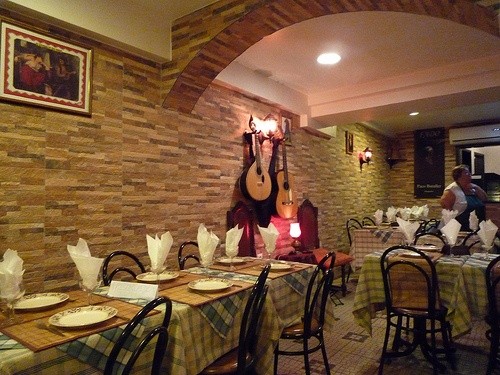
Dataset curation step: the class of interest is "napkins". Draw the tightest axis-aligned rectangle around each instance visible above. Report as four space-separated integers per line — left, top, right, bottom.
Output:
371 203 499 249
225 223 244 256
257 222 279 252
145 231 173 271
66 239 105 290
197 223 220 266
0 248 25 299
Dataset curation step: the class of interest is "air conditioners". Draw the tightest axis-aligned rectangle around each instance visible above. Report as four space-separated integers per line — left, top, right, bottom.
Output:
448 124 500 146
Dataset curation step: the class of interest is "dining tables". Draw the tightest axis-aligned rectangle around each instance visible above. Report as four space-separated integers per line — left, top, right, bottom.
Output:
0 222 500 375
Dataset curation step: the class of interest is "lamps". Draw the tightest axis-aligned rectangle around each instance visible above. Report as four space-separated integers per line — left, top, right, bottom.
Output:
289 223 305 254
359 147 373 170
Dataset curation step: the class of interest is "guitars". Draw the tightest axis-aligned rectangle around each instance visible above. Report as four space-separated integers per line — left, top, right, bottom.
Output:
238 122 273 205
271 124 299 221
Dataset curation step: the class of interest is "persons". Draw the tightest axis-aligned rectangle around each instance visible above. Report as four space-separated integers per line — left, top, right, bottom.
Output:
439 165 487 232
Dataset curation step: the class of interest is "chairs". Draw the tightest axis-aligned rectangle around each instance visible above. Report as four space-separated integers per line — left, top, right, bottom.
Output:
101 214 500 375
296 200 353 297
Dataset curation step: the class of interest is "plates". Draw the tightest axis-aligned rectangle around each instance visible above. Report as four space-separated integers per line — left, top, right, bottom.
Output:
7 292 70 309
261 263 291 270
216 256 244 263
187 277 234 292
48 305 119 327
400 244 444 258
136 271 179 282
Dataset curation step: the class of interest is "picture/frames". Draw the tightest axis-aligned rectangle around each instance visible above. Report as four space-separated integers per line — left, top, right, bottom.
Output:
346 130 354 155
279 113 293 146
0 17 94 119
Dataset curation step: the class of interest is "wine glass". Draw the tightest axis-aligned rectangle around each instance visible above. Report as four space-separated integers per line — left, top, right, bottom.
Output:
445 239 458 259
480 240 494 261
403 234 415 250
